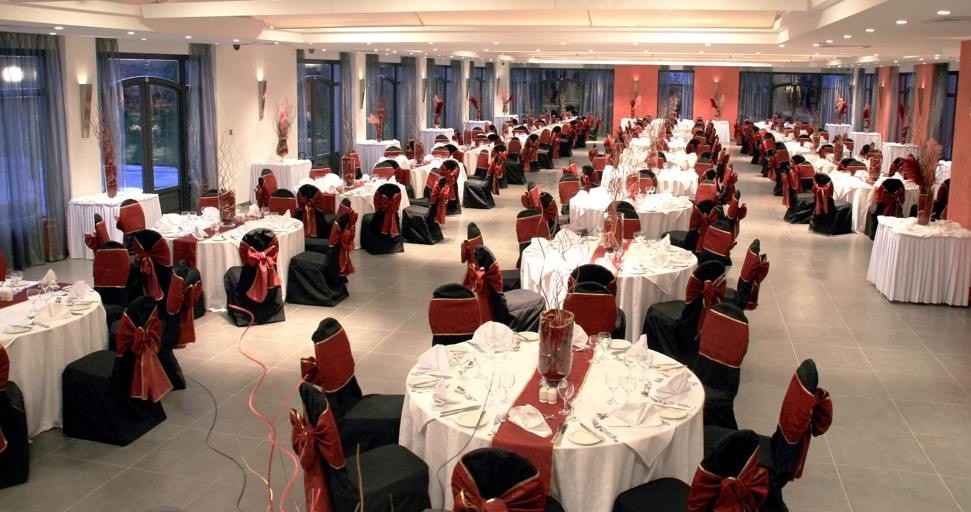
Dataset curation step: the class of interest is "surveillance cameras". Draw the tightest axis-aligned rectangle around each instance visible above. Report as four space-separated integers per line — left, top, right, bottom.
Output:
233 45 240 50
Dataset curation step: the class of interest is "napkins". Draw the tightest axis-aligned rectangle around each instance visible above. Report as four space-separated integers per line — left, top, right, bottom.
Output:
623 334 651 361
655 370 692 394
66 280 90 300
415 344 451 376
472 321 515 350
572 321 588 346
34 302 70 324
607 399 664 428
432 377 463 406
39 268 57 287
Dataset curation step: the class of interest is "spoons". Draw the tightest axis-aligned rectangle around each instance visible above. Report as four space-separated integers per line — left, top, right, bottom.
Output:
457 360 474 376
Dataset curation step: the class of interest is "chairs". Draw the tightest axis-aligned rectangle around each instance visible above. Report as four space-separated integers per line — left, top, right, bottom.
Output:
724 237 769 312
683 303 750 429
704 358 833 511
463 245 547 332
428 282 483 348
285 212 356 307
83 213 110 248
641 261 728 367
105 228 187 391
562 281 618 339
300 316 406 459
611 429 768 512
92 240 131 313
226 109 749 280
0 346 31 490
289 381 433 512
565 263 627 340
59 295 174 448
733 113 952 243
114 199 146 235
450 446 567 512
223 228 287 328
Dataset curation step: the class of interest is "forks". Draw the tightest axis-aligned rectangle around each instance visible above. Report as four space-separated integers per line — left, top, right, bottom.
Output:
592 418 618 438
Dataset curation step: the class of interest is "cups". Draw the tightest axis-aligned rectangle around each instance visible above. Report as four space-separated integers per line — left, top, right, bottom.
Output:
539 387 549 403
549 389 558 404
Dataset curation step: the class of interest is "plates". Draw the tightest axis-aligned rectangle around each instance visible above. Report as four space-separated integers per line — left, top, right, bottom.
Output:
409 376 439 388
445 343 468 357
69 305 91 311
609 338 632 349
516 331 540 341
455 411 489 428
6 327 31 333
568 428 603 445
660 407 688 418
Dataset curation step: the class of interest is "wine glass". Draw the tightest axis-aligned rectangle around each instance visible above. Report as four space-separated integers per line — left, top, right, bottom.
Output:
589 334 601 364
623 375 636 400
484 354 518 404
646 186 656 198
5 271 23 295
558 380 575 414
604 374 623 405
597 332 612 352
182 210 197 226
26 289 40 312
614 247 624 260
568 395 584 423
254 207 278 226
634 231 646 245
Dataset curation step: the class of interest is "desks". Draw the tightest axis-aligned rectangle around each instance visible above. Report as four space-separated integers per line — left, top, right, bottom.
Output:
520 235 698 342
1 280 109 440
398 333 706 512
151 211 305 312
865 215 971 306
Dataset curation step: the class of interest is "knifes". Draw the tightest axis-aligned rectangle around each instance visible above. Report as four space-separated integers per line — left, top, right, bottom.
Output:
581 423 605 440
